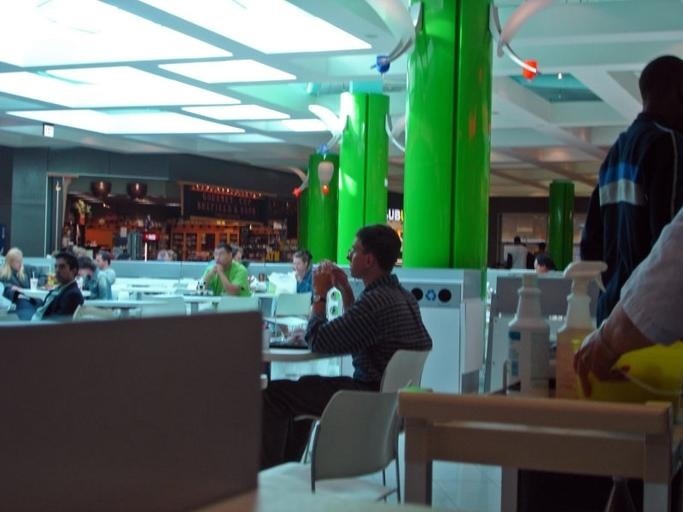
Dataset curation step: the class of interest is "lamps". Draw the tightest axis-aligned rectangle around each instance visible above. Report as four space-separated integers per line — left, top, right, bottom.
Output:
385 112 405 153
308 103 347 160
489 0 552 80
370 1 423 75
290 167 308 197
317 162 334 195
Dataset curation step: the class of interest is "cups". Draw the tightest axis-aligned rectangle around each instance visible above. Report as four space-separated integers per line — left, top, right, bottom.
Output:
30 278 38 291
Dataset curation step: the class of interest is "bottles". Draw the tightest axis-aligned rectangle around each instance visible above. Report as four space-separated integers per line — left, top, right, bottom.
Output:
556 278 597 400
258 297 272 316
47 276 54 291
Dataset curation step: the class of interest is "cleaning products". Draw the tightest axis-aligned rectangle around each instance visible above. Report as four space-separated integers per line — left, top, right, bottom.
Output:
551 253 606 398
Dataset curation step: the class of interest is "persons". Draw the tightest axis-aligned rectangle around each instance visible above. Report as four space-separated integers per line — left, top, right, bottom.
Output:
573 206 683 397
532 253 558 275
505 235 546 270
0 223 117 322
579 54 683 331
259 223 433 472
230 244 244 263
201 242 252 298
291 248 315 295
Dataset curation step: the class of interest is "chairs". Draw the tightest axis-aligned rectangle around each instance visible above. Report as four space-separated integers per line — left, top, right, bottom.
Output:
398 390 672 512
